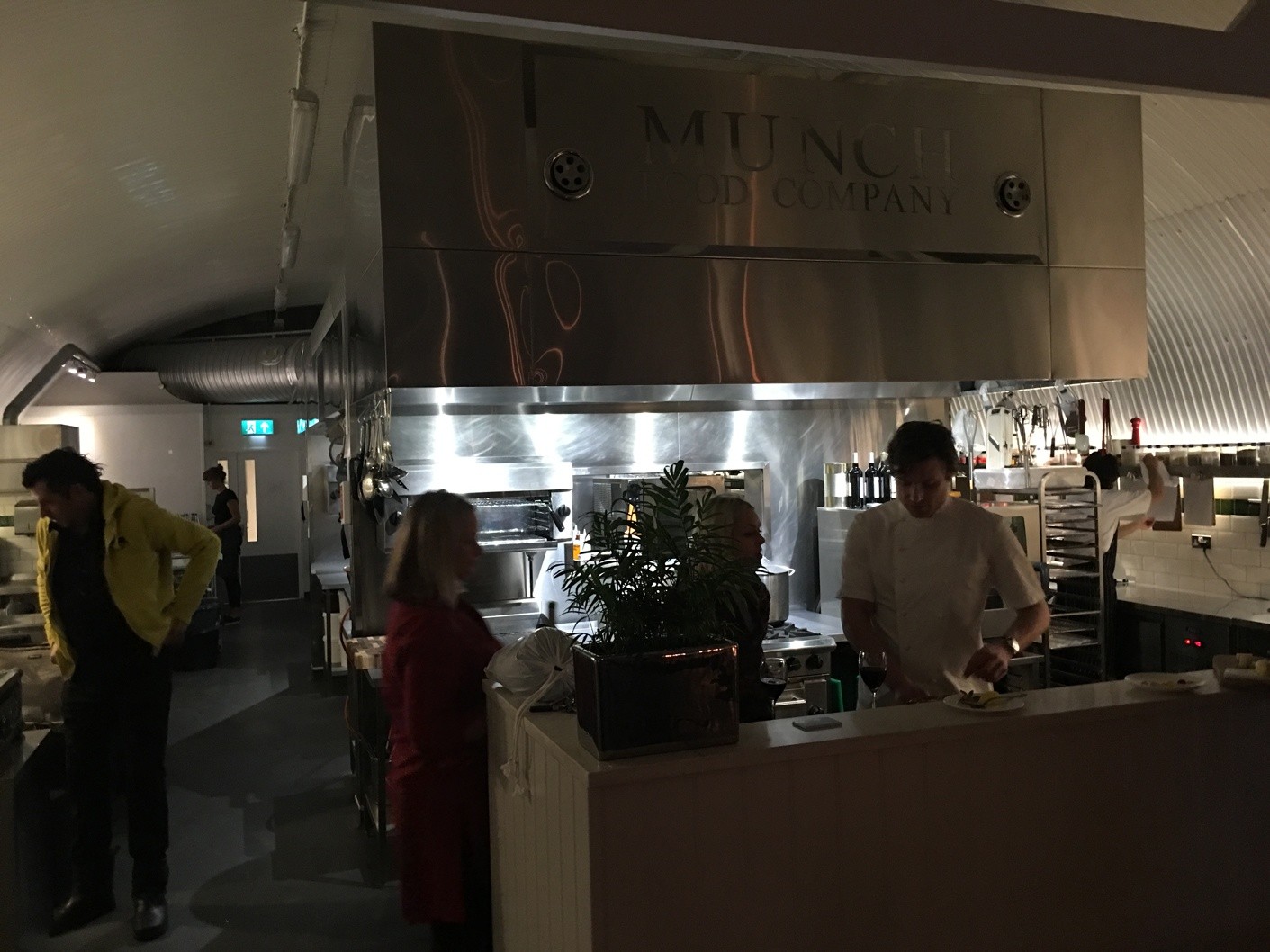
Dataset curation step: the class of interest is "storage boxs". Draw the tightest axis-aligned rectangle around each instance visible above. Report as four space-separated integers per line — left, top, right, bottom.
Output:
1028 464 1087 488
973 468 1026 490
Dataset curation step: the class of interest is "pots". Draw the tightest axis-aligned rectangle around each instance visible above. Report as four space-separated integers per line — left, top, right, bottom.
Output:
756 565 795 622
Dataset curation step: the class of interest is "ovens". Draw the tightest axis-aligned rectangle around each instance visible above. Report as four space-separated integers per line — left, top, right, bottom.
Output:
771 680 828 719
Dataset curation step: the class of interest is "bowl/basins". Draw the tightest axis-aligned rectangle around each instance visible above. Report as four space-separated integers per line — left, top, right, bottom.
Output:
343 565 351 584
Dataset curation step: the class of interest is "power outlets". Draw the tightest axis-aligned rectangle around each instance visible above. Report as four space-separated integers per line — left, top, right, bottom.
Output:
1191 535 1212 549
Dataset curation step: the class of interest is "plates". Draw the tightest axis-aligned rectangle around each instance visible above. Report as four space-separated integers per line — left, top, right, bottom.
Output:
943 693 1024 712
1124 672 1211 692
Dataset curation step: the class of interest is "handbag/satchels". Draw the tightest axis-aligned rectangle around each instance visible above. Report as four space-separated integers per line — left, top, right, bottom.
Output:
484 626 583 796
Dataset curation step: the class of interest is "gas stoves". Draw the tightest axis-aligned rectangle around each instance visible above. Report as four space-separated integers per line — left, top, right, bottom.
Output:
761 622 837 678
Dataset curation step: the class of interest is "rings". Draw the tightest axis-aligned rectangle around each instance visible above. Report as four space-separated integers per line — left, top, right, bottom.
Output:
909 699 912 702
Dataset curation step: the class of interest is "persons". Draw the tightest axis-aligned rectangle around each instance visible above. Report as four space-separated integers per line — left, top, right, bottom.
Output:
1010 449 1034 466
202 464 243 626
22 447 222 942
380 491 503 952
1052 449 1165 686
835 421 1050 711
668 495 776 723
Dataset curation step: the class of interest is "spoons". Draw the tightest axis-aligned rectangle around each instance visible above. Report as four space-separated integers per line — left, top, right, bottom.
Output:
967 693 1028 709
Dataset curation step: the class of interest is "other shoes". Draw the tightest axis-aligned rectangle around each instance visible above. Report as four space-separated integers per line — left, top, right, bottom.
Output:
133 892 168 940
220 615 240 627
52 887 115 929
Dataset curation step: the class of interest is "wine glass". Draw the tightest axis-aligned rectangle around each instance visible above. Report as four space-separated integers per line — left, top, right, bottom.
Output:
858 649 888 710
759 657 787 720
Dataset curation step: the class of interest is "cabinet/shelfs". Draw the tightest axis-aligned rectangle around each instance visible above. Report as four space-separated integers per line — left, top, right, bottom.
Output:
970 471 1107 690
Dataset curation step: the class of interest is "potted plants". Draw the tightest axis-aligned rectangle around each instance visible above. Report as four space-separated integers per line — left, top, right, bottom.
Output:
546 459 776 760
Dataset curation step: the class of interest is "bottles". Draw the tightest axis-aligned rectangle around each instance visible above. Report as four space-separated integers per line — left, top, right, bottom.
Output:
848 452 896 510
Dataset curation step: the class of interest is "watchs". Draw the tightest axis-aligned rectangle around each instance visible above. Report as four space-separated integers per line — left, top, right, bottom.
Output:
1003 635 1022 657
